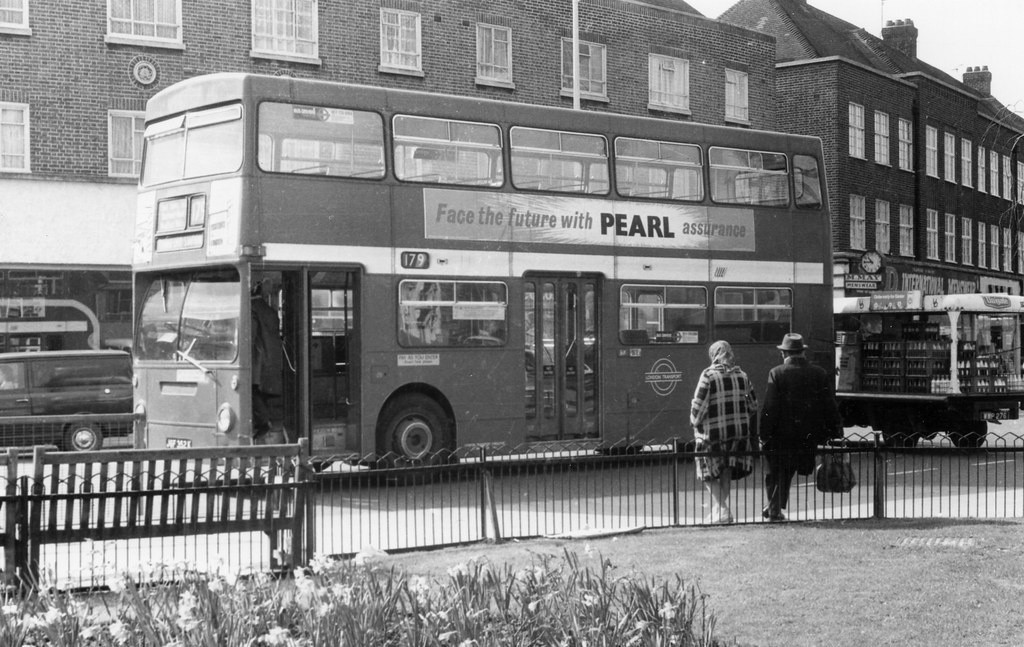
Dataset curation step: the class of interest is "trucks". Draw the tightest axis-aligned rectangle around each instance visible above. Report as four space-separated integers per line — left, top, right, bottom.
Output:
829 294 1024 451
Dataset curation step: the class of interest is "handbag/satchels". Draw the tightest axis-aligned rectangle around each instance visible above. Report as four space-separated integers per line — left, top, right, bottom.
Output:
815 451 857 493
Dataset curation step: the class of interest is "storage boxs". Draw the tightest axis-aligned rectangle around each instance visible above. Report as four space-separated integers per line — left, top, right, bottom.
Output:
862 341 1024 393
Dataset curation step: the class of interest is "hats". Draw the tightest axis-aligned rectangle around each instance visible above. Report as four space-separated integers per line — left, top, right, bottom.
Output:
776 333 809 351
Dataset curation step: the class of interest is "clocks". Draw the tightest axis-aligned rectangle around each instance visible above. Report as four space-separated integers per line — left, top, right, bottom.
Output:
860 251 886 274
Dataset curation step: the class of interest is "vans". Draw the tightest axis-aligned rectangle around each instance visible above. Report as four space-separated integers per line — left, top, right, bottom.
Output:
0 349 132 451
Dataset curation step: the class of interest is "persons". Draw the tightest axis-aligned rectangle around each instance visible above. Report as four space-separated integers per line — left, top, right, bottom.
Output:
759 333 828 521
689 339 757 524
248 278 285 445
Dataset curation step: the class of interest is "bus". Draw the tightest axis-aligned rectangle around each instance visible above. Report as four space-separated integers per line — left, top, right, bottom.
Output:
132 71 835 483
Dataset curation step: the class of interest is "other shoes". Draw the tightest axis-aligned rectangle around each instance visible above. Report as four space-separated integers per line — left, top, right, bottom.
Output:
253 422 272 440
762 503 784 520
709 506 733 524
